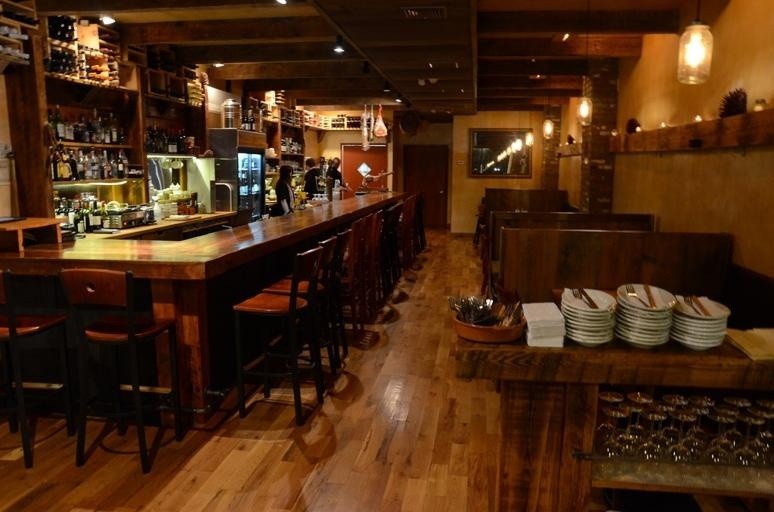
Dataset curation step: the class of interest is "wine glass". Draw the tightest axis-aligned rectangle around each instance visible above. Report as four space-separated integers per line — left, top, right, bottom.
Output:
312 193 328 202
595 391 774 492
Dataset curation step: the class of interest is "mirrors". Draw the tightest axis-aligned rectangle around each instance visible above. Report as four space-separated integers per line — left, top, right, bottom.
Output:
469 127 534 179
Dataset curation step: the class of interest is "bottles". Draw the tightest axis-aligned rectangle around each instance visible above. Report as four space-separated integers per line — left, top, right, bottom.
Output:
243 105 256 131
47 103 119 145
331 116 362 128
53 192 103 234
145 121 187 152
260 103 303 127
48 145 129 182
44 15 120 82
186 82 206 103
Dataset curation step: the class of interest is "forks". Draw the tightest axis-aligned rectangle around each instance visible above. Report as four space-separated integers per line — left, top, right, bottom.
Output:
445 290 496 323
684 294 702 316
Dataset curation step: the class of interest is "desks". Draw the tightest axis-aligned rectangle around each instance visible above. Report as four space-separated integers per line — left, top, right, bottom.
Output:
93 208 237 239
453 328 773 512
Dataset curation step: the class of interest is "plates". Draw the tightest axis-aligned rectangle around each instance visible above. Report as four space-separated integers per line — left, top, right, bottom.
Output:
615 284 677 350
559 288 617 347
670 299 732 350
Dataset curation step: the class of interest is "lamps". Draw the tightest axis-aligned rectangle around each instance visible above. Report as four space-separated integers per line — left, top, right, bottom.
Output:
526 131 534 147
575 94 594 126
543 118 555 139
676 9 714 88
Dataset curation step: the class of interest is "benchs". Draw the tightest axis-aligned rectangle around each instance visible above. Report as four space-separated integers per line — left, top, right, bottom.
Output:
500 229 735 329
474 186 654 290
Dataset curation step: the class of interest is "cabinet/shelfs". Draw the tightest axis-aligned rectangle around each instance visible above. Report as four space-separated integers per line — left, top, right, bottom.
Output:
275 103 305 170
0 17 149 251
141 51 207 156
304 109 383 132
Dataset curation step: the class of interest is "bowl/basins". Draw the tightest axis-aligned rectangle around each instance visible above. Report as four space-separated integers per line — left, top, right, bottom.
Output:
451 313 526 344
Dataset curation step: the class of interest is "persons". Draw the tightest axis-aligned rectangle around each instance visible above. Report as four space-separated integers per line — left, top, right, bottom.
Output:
326 158 352 200
303 158 323 200
275 165 296 216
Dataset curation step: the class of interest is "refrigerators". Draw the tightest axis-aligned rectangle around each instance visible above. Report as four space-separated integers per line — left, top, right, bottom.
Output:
206 127 266 227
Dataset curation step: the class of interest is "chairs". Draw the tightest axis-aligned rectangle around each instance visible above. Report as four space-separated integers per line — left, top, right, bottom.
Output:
57 267 187 473
0 268 79 469
